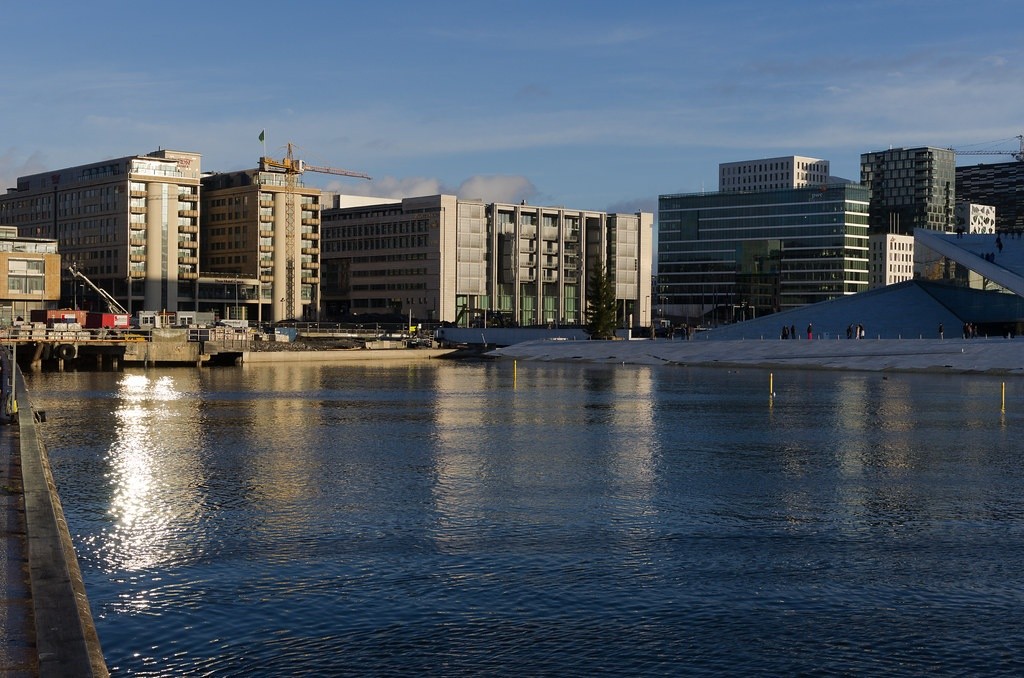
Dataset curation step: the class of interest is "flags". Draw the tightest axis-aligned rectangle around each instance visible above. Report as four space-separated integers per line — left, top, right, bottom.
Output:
259 130 264 142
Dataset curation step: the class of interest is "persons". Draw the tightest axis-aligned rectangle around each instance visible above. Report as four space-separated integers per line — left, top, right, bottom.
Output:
679 326 690 340
847 323 864 339
650 325 654 339
791 325 795 338
939 323 944 339
782 326 789 339
1002 324 1015 338
1011 229 1015 239
1018 229 1021 237
985 252 995 262
963 322 978 339
955 227 963 239
665 327 674 340
807 323 813 339
996 229 1003 253
1004 229 1008 238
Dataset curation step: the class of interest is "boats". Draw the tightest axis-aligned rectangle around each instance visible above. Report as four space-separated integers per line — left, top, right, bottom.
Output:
433 305 594 350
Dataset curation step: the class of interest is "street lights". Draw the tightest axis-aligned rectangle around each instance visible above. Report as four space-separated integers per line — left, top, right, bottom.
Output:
644 295 651 328
72 261 78 311
235 274 240 321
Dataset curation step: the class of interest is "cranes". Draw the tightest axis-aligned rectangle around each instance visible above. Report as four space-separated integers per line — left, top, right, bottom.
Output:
256 141 373 321
